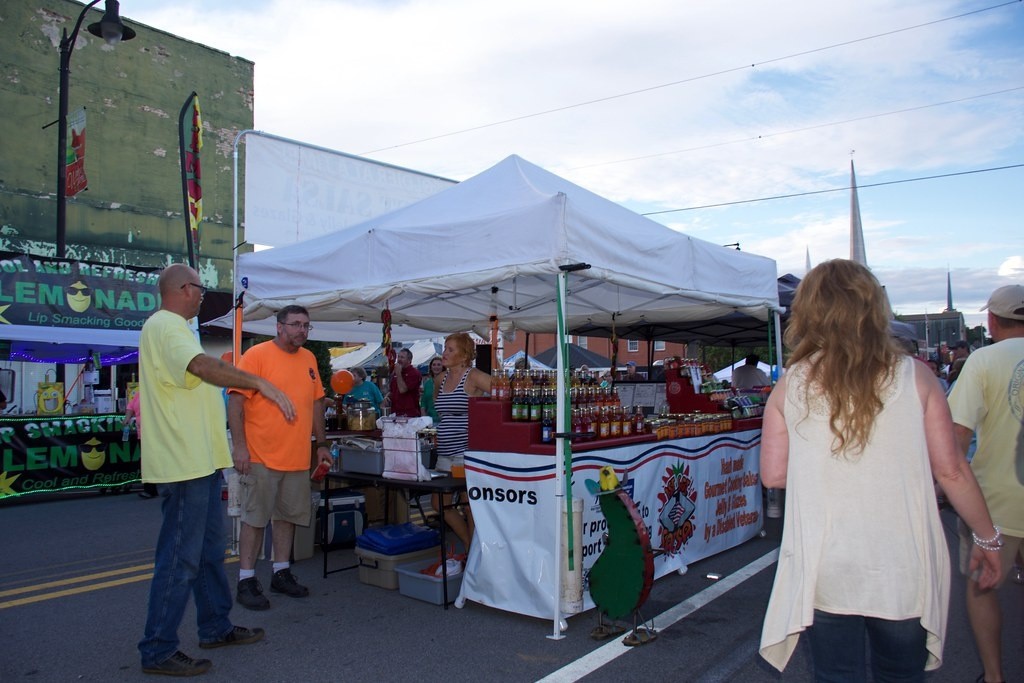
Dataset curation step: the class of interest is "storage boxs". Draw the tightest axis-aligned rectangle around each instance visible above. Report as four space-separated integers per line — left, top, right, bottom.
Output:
317 487 367 552
354 541 448 590
339 445 383 475
393 555 463 606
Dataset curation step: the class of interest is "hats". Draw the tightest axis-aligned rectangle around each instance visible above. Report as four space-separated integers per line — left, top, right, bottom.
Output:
979 284 1024 320
948 340 969 350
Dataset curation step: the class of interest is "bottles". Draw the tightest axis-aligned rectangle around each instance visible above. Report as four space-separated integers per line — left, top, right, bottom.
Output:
310 460 330 483
329 441 340 473
490 368 632 445
329 394 372 430
121 423 129 441
634 404 644 435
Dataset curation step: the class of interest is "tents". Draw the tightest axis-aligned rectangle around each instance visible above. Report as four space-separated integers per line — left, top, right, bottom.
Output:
570 274 917 380
228 154 785 639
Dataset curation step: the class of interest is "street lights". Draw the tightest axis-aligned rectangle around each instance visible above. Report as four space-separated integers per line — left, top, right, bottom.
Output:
937 326 956 373
54 0 136 417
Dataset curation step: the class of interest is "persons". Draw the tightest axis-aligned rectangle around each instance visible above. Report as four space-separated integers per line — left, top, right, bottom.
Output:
893 333 988 498
760 259 1002 683
122 263 529 674
732 354 771 400
947 285 1024 683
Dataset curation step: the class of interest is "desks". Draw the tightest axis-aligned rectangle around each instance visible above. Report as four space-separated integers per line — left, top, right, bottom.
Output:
322 472 469 611
455 396 769 630
312 429 382 469
0 414 144 507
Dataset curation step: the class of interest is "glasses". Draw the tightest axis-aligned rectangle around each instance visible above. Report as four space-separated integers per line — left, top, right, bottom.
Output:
180 283 207 295
278 321 313 330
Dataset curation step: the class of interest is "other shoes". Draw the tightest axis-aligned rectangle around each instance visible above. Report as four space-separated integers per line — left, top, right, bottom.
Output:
236 575 270 610
270 568 308 597
143 651 212 674
137 491 159 499
199 625 266 648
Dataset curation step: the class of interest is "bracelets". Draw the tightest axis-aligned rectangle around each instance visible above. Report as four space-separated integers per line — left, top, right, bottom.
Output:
971 524 1004 550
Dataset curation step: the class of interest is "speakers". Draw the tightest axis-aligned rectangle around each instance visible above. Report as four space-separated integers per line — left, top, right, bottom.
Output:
476 344 491 375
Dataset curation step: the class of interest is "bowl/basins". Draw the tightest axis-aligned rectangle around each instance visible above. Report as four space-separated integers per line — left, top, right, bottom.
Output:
345 407 377 430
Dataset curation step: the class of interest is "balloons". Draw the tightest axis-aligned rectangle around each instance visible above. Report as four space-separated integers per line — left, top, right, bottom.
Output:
331 370 355 394
